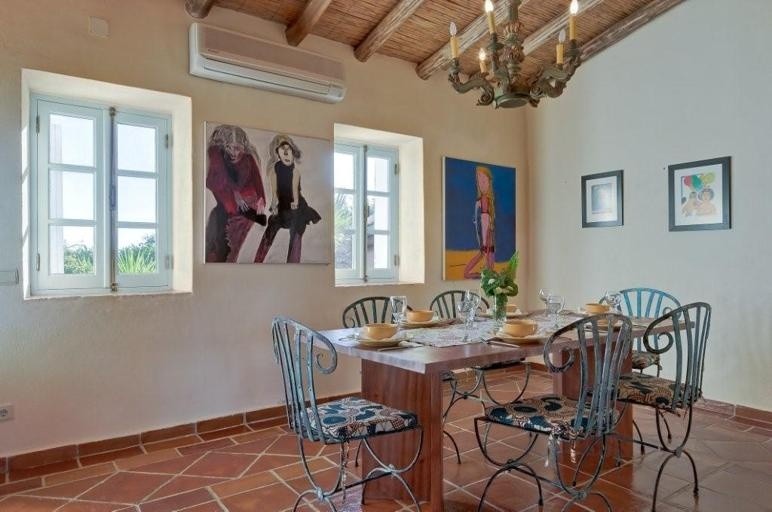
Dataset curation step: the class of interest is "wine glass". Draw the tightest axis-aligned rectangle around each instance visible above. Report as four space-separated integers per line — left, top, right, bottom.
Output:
538 289 565 331
603 289 624 319
456 286 481 342
390 296 407 339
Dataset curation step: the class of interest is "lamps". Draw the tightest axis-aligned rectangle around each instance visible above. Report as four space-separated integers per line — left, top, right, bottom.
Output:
447 0 581 109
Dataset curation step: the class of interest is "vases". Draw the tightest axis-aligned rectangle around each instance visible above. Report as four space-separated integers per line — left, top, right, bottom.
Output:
494 295 506 322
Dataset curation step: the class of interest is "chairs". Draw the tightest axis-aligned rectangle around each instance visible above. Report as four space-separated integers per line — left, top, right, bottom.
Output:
271 314 424 512
474 314 634 512
430 291 532 455
599 287 682 454
573 302 712 512
342 297 461 467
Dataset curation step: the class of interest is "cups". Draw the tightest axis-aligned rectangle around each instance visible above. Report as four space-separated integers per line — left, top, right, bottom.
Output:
406 310 435 321
363 324 397 339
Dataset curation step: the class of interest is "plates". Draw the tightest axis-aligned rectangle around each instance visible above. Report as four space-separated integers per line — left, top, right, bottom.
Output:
501 337 546 344
393 318 444 327
353 337 404 348
586 323 639 331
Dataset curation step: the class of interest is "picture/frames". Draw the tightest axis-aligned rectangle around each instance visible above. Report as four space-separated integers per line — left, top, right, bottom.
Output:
581 169 623 228
668 156 730 232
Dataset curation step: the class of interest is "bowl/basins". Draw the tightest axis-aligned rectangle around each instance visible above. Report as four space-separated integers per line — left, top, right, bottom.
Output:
504 305 517 312
504 320 538 337
585 303 604 314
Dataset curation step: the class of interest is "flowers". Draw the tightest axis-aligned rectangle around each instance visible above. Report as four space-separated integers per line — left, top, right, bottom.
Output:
480 252 520 319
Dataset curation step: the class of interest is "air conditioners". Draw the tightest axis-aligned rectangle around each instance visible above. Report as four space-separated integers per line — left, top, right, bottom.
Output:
188 22 347 104
